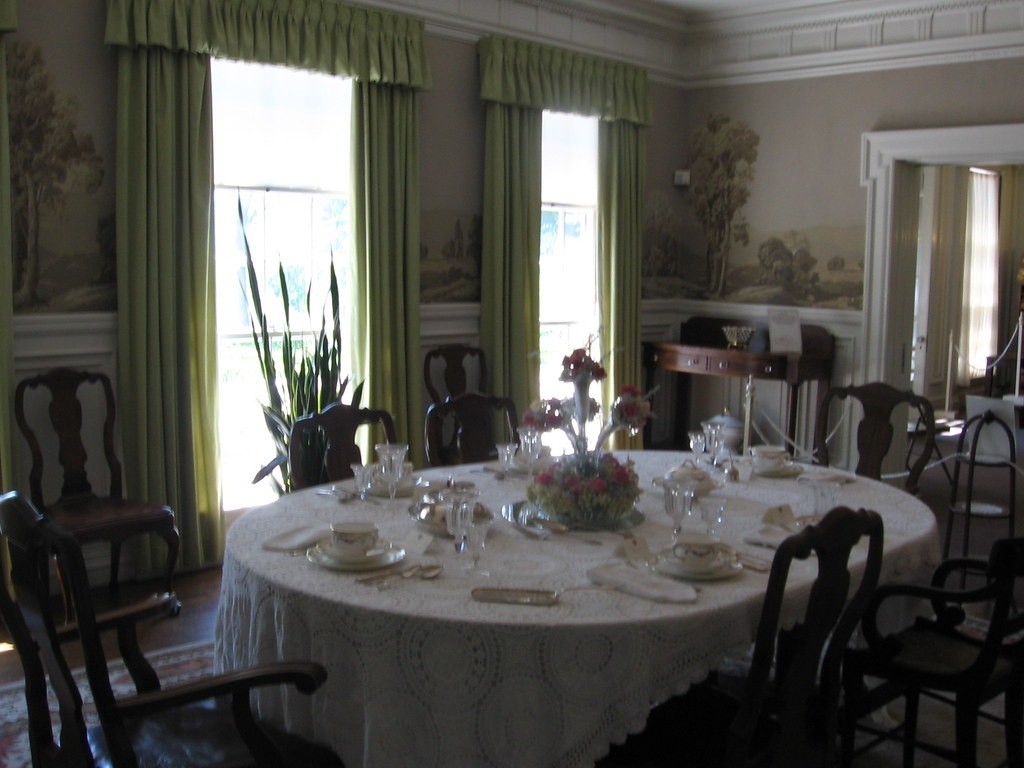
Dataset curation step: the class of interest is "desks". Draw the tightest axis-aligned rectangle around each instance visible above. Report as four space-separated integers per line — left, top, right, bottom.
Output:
641 318 836 453
220 450 943 767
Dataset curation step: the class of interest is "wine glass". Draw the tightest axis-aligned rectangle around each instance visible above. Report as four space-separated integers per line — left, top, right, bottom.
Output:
689 430 705 465
489 444 518 488
438 488 490 579
349 463 376 501
516 426 542 483
660 480 695 544
701 422 727 473
697 497 726 533
374 443 409 499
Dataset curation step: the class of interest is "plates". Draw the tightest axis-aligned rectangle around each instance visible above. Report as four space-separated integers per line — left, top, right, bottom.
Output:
315 538 392 564
307 544 405 570
753 465 804 477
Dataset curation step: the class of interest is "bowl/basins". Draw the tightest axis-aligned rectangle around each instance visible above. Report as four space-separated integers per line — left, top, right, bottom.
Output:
332 527 378 556
407 505 494 538
651 477 723 499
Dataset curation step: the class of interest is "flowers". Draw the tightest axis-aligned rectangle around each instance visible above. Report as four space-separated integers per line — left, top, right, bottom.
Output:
523 349 654 499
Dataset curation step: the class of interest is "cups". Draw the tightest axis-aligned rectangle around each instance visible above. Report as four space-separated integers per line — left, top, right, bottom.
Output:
751 446 790 471
722 326 754 351
647 547 742 580
795 480 840 529
708 406 744 448
671 534 720 565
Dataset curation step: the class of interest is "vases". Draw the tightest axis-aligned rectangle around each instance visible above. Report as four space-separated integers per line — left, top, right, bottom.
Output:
528 490 636 531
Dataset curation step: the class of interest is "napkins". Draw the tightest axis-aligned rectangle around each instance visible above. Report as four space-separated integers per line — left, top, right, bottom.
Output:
261 519 333 553
736 522 794 546
581 557 699 605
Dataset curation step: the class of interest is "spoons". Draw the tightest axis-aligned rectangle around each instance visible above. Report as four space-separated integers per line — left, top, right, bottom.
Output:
355 564 443 588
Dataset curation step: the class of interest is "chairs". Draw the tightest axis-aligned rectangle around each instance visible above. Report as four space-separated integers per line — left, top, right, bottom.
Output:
644 506 886 767
0 496 346 768
814 380 935 497
14 367 184 627
905 407 952 479
849 536 1024 768
423 392 523 469
290 402 396 492
423 341 487 407
